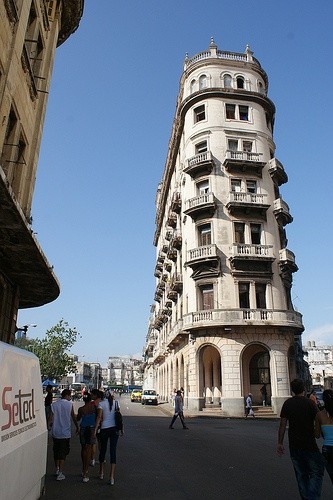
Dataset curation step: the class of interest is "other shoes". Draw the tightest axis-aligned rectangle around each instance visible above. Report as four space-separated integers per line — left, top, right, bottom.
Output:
169 426 175 429
56 474 66 480
108 479 114 485
83 477 90 483
56 469 60 475
183 426 190 429
99 473 104 479
90 459 96 467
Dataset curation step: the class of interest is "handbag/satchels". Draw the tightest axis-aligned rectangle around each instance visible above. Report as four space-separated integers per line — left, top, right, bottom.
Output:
250 409 255 416
115 401 123 430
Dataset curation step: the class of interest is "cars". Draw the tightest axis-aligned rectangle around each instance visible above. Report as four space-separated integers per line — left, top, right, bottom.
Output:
129 389 142 402
140 388 159 405
314 392 325 407
313 388 321 395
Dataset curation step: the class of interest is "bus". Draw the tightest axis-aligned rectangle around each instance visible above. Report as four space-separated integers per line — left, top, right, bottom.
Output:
70 382 87 397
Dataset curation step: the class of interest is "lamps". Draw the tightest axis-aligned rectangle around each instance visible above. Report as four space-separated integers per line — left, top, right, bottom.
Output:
15 324 28 335
225 328 232 330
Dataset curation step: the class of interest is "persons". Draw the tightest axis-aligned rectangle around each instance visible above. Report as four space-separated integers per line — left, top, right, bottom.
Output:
93 389 124 485
75 391 98 482
277 379 324 500
47 389 78 481
315 389 333 486
260 382 269 406
91 389 107 466
168 391 190 429
245 393 256 420
310 392 318 406
45 384 53 430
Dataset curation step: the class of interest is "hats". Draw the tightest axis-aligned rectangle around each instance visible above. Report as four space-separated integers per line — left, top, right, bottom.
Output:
249 393 253 395
